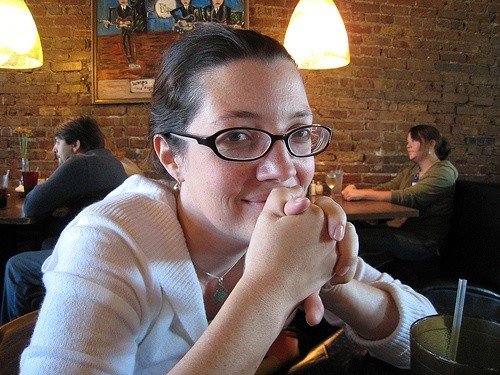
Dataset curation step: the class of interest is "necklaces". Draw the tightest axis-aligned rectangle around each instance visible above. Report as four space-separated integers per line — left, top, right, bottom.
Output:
196 250 246 306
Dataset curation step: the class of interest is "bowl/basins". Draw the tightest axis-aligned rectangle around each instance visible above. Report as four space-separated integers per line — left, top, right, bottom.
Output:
16 191 24 196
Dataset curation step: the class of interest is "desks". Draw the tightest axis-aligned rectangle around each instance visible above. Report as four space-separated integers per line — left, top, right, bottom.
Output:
0 188 54 225
328 195 420 221
281 285 500 375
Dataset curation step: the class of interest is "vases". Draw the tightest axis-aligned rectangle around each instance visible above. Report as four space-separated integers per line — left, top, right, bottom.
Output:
22 159 29 173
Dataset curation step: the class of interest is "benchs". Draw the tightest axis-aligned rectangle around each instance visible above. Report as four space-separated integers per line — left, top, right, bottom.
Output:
377 177 500 296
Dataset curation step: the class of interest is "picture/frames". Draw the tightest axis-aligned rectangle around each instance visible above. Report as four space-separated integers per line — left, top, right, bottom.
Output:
91 0 249 105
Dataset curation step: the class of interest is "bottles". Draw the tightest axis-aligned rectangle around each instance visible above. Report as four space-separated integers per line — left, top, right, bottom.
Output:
310 180 323 195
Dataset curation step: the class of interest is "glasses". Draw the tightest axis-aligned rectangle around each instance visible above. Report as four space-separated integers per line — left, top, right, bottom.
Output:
167 122 334 164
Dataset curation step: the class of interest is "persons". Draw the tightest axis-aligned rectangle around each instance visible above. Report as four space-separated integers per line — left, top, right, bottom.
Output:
18 24 449 375
342 125 460 268
0 116 129 326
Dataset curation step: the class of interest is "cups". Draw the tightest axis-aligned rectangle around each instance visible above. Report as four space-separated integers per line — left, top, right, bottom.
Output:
409 313 500 375
330 170 343 196
22 171 38 196
38 178 45 185
0 169 9 209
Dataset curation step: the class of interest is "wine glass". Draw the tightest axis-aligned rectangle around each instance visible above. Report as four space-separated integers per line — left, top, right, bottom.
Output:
326 173 336 200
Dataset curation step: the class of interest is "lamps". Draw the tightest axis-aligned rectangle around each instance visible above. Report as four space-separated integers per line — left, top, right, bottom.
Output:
283 0 350 70
0 0 44 69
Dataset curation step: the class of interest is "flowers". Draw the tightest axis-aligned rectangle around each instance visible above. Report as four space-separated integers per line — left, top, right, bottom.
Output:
12 127 33 160
